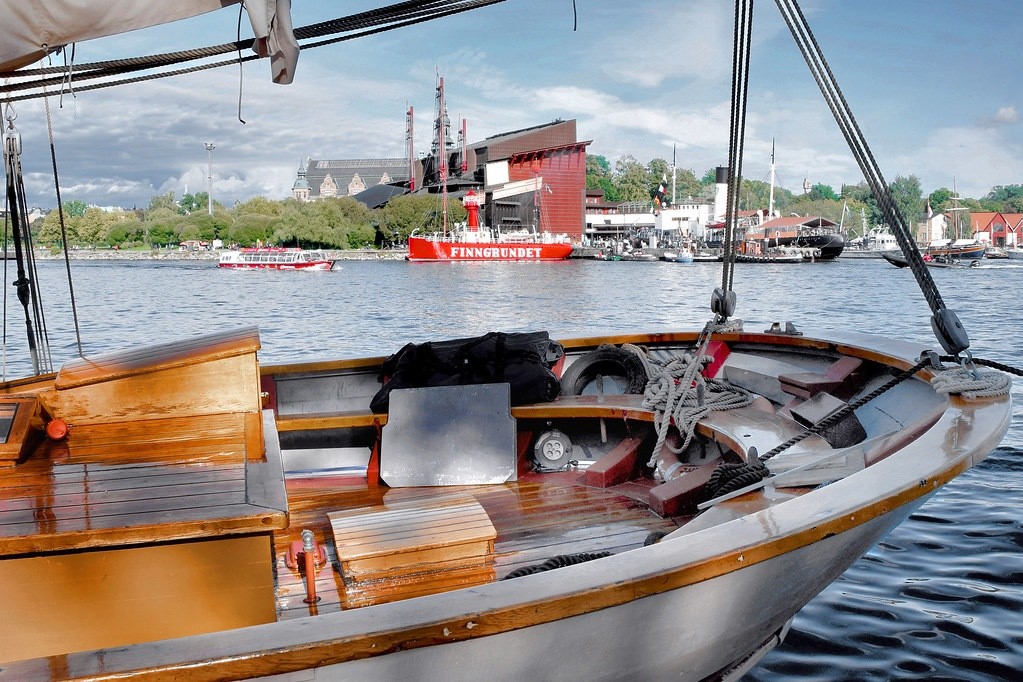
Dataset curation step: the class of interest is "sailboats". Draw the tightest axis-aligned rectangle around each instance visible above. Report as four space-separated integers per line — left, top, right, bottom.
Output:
405 78 1009 271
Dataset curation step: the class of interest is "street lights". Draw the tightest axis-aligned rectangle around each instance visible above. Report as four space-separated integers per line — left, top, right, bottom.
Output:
204 143 217 214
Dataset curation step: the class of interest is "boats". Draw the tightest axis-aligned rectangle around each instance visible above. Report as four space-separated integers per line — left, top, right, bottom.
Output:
217 244 336 271
0 1 1018 682
